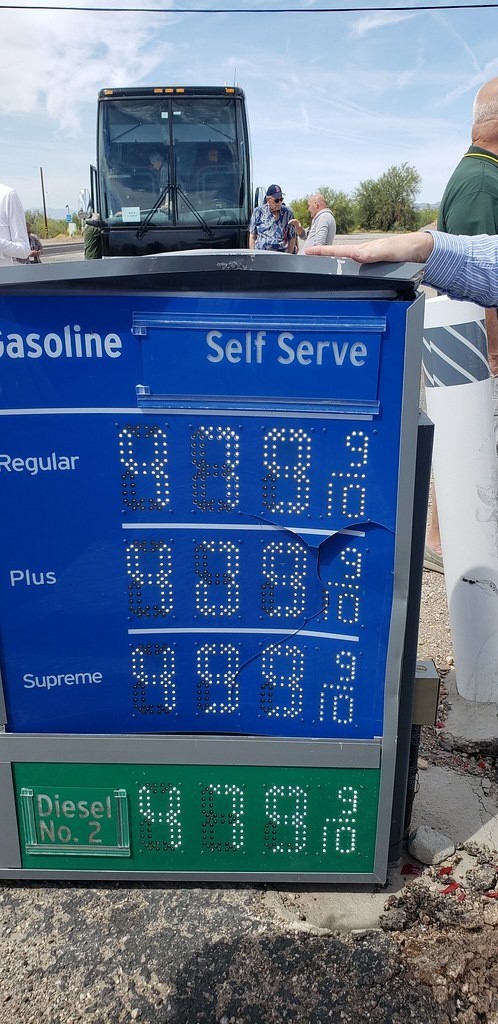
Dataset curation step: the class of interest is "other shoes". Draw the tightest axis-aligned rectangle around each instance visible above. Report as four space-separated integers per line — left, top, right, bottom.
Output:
422 544 444 574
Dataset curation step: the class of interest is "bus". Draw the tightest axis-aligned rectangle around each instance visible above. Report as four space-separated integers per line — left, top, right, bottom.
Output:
78 81 257 256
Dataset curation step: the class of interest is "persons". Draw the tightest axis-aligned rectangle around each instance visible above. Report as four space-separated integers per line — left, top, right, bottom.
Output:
304 229 496 307
196 148 228 201
14 222 43 263
249 185 297 253
83 213 102 258
177 142 197 188
288 195 336 255
423 76 498 578
149 152 186 211
0 183 31 267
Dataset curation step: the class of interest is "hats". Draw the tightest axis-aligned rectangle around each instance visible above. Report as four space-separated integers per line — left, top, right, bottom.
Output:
267 184 286 198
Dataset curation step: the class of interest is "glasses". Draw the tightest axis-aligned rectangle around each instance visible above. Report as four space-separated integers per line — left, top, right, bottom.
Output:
269 197 284 203
28 231 31 234
